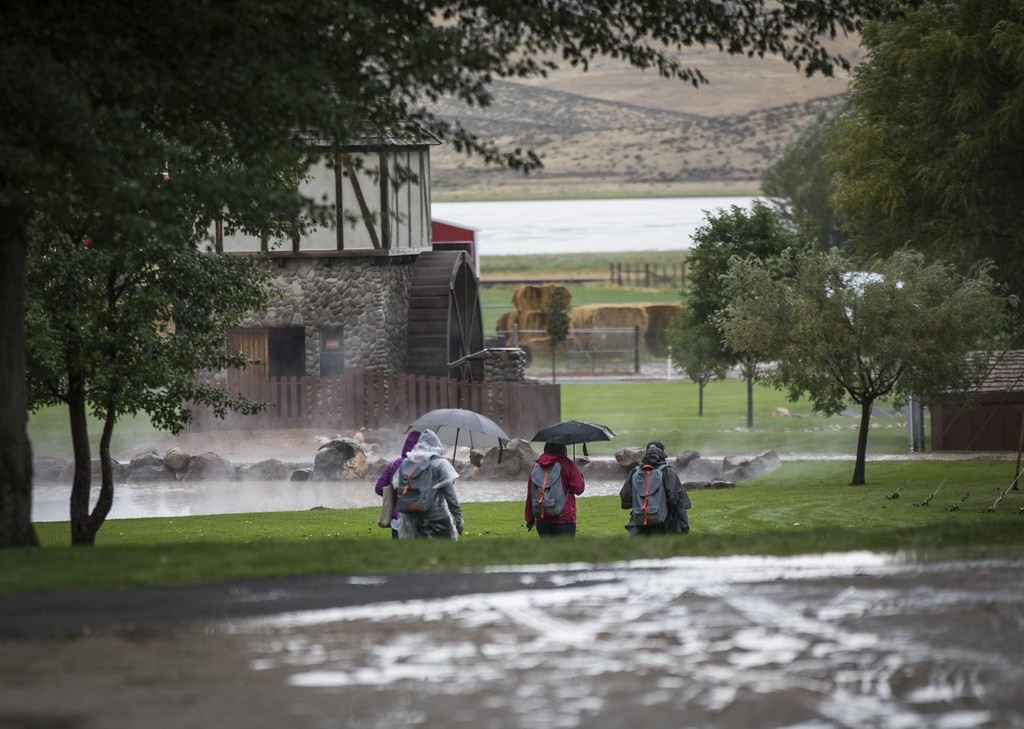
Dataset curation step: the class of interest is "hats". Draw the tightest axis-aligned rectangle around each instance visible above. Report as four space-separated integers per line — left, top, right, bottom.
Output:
647 441 665 450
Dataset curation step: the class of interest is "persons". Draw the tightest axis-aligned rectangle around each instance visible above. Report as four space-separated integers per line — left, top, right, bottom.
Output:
524 443 585 536
375 431 421 538
620 440 691 536
390 428 465 539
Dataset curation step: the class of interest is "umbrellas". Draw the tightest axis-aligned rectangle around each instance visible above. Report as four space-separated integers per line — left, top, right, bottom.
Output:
530 419 616 462
403 409 511 467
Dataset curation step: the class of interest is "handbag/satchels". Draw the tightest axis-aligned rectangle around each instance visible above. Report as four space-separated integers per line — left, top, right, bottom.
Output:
378 476 394 528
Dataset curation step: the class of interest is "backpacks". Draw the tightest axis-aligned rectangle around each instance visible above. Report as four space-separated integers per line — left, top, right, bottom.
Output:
632 465 671 526
396 452 445 513
530 461 570 518
